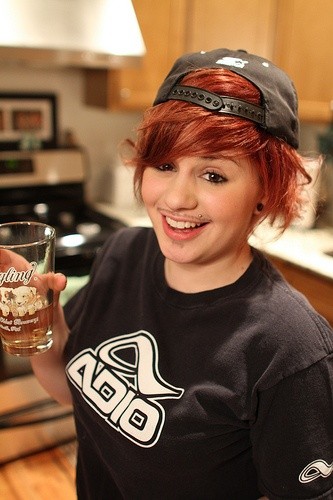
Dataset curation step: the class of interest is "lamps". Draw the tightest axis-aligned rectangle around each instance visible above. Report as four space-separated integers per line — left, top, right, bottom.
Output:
0 0 147 56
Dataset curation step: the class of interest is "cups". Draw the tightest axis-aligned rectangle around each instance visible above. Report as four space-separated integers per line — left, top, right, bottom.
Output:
1 222 58 357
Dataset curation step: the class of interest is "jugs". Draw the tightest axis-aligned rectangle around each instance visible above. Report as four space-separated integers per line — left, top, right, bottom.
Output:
287 151 324 231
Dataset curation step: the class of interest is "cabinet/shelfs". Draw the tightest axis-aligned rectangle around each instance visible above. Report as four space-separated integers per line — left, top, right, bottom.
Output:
80 1 331 118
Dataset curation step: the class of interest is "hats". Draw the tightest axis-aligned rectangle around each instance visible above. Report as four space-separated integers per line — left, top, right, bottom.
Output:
153 48 300 150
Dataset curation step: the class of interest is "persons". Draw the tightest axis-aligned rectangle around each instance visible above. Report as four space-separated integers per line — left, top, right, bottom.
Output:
0 46 333 500
